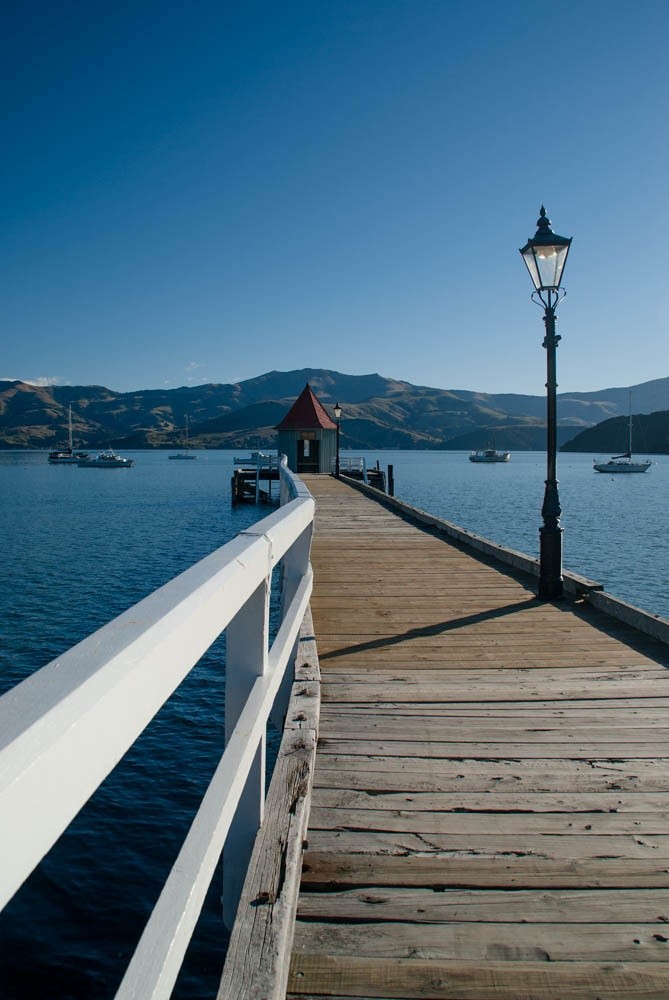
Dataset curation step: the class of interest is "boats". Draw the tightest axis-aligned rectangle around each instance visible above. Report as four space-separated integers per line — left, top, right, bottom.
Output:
469 448 511 462
233 452 275 464
593 458 653 473
169 453 196 459
78 454 133 467
48 448 88 463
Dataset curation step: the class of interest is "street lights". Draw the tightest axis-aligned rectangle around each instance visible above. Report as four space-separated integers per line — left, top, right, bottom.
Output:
333 401 341 479
518 201 575 603
